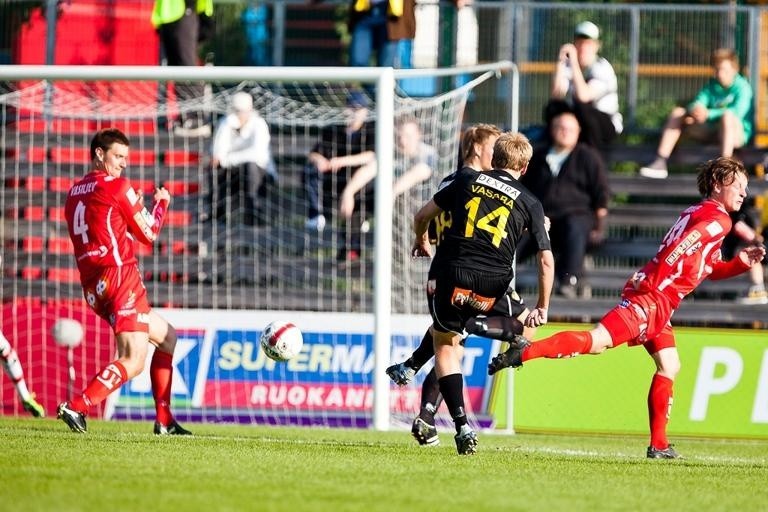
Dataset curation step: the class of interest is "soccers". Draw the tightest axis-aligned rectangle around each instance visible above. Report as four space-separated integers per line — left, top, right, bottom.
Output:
260 319 305 361
52 318 84 347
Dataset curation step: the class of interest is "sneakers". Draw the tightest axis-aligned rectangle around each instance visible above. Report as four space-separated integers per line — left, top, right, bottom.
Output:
202 210 263 229
734 284 767 306
386 362 416 388
454 422 479 458
21 397 45 419
153 419 193 436
487 341 525 377
411 416 441 451
56 400 88 434
639 156 669 180
646 442 688 462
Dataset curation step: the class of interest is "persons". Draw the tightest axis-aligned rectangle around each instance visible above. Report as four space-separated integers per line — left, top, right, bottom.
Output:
1 335 47 417
326 113 436 269
383 120 537 446
193 91 278 231
152 0 216 137
346 0 417 124
488 157 766 459
56 129 195 436
637 47 754 180
541 22 624 148
301 89 374 231
409 129 557 458
728 189 768 304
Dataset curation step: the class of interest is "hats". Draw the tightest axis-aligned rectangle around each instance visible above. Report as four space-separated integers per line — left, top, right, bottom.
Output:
573 20 600 40
230 91 253 111
345 90 369 108
